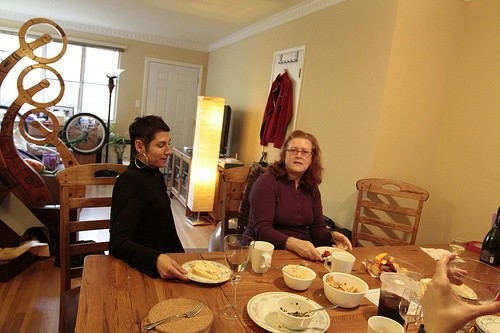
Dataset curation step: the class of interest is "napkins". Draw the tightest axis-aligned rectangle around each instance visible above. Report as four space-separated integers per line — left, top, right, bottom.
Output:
366 288 423 315
420 247 466 263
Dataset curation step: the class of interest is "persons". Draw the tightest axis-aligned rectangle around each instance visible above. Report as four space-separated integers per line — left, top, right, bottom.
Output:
109 116 187 282
240 131 354 261
418 253 500 333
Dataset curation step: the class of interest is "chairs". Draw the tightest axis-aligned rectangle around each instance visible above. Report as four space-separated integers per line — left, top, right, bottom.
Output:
350 178 430 247
221 166 251 251
56 164 128 333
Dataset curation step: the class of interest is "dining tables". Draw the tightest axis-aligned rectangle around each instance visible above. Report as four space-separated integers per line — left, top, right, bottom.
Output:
74 243 500 333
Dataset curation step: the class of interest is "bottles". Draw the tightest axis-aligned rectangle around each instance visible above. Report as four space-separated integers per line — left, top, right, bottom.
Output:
479 205 500 267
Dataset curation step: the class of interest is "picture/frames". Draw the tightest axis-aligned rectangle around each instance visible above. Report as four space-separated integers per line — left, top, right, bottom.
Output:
37 107 74 123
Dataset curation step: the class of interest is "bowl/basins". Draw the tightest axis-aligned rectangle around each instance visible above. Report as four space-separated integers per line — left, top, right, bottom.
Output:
282 265 316 291
323 272 369 308
367 315 404 333
275 296 316 330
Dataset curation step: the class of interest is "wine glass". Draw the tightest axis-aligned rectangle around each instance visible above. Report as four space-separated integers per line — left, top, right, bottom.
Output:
219 234 255 320
398 288 422 333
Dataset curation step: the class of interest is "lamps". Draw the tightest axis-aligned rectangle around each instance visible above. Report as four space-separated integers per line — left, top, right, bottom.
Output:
104 68 126 164
185 96 225 226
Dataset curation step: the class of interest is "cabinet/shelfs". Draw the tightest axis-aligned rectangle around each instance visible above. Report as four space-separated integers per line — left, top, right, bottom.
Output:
168 147 245 227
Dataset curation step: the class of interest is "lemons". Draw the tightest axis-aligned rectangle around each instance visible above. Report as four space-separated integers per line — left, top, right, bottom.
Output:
375 252 387 264
381 256 399 270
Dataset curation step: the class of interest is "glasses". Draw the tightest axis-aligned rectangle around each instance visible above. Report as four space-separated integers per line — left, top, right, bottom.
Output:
286 149 313 158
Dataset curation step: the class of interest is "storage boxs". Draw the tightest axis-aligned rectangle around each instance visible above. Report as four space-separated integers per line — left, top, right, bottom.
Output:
44 154 57 170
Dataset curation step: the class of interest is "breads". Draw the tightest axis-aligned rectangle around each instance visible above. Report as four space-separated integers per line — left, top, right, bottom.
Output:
191 260 220 280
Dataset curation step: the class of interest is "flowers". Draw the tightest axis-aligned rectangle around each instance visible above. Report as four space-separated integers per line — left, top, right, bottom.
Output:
105 122 130 159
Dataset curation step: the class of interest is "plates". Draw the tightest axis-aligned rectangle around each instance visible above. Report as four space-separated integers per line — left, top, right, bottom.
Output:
181 260 232 284
474 315 500 333
247 292 331 333
316 247 345 262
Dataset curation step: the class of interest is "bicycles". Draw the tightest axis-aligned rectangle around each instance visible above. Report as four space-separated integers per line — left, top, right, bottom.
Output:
0 104 107 155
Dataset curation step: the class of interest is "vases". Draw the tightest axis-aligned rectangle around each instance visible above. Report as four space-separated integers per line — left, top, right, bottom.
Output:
118 153 122 164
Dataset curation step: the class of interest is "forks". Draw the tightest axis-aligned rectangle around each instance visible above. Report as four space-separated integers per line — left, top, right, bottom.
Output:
144 302 205 328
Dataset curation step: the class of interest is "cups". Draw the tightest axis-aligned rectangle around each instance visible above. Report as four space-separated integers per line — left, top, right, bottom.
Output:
324 251 356 274
449 236 467 256
249 241 274 274
447 257 500 333
376 272 417 326
